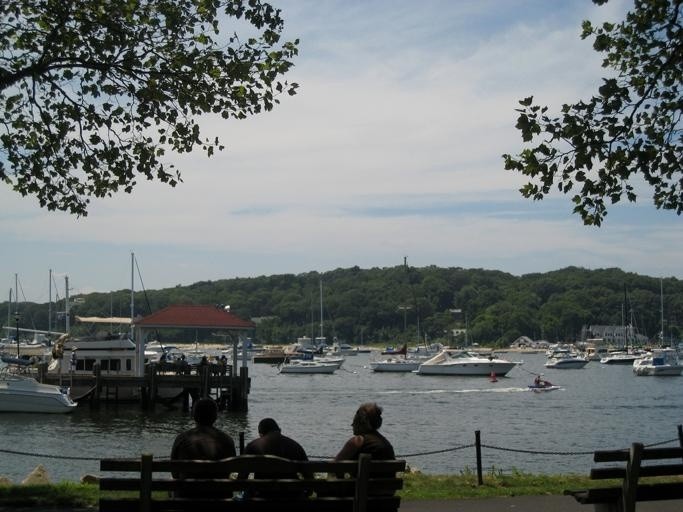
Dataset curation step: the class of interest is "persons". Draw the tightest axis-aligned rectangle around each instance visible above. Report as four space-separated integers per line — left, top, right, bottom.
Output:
534 376 544 387
326 402 395 498
235 418 315 498
68 346 78 373
170 398 237 499
160 353 227 376
284 357 289 363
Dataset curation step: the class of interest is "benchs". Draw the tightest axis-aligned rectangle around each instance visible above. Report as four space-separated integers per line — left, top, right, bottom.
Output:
99 453 405 512
154 363 232 376
563 442 683 512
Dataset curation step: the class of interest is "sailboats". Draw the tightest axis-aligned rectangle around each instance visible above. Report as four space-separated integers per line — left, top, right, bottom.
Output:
0 250 213 415
543 275 682 378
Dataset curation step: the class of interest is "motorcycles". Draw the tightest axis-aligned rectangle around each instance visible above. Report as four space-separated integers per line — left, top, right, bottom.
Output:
526 383 562 393
